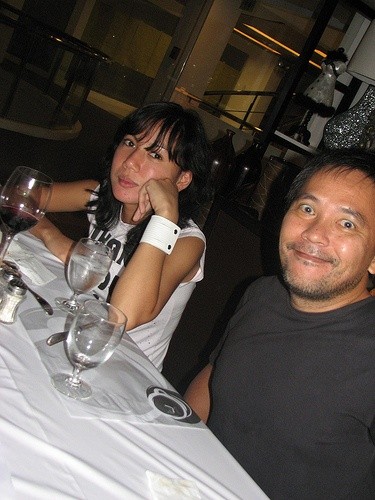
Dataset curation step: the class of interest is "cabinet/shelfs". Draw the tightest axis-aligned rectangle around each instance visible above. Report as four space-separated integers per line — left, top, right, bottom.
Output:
202 0 375 247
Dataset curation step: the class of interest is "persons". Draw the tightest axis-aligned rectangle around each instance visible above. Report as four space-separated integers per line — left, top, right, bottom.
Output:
182 146 375 500
0 100 212 375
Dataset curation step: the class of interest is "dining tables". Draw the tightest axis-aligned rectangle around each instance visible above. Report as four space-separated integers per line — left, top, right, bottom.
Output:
0 231 271 500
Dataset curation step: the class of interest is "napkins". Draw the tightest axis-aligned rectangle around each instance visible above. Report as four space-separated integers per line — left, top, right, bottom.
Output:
5 238 57 286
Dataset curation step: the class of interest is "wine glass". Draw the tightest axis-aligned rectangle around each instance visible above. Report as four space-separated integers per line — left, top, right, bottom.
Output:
50 300 129 400
55 236 114 317
0 165 54 274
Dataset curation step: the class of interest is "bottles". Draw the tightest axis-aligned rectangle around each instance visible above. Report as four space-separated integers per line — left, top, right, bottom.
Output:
223 137 264 203
0 279 28 324
205 129 236 196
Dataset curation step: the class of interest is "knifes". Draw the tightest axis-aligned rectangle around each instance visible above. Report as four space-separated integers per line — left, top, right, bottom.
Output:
46 319 109 347
26 285 54 316
